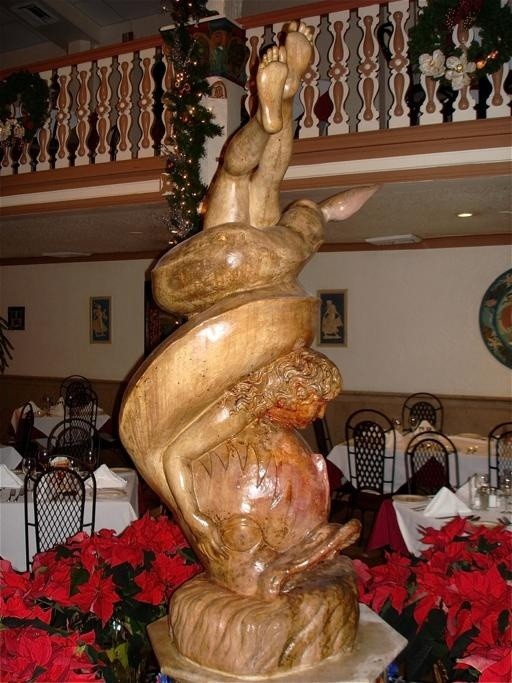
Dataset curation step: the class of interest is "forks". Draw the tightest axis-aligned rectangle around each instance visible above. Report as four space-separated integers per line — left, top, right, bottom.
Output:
8 486 24 503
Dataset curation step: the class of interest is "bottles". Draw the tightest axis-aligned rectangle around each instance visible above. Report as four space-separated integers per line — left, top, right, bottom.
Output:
471 476 512 510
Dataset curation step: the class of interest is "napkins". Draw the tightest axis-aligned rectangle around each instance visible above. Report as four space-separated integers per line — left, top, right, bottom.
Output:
424 486 472 517
456 477 485 502
83 464 126 490
414 421 435 434
1 463 24 488
362 425 387 438
32 474 51 502
25 400 40 413
53 404 66 417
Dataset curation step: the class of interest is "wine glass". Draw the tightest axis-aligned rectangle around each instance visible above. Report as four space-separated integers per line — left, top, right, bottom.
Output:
83 448 97 478
22 448 49 491
392 417 418 432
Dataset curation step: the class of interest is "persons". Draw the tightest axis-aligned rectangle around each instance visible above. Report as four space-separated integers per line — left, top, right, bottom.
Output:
161 20 341 564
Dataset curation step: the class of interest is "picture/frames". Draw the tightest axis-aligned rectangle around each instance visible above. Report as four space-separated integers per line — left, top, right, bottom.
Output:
315 289 349 349
89 295 112 344
7 307 25 329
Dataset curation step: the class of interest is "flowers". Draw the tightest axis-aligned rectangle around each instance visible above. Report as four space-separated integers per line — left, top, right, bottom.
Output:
0 516 512 683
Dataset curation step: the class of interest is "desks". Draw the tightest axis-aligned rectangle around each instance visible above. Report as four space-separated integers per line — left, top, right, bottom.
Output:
33 412 108 439
368 493 512 554
1 468 138 571
326 431 510 494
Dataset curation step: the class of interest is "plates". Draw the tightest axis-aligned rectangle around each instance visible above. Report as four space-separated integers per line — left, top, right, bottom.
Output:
109 467 132 475
468 521 499 530
91 487 126 500
392 494 427 504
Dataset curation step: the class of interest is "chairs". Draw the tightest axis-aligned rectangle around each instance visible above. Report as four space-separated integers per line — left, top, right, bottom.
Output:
98 396 122 465
402 392 444 431
66 387 98 433
336 408 396 522
486 423 511 489
404 429 461 497
59 375 91 403
25 454 96 573
12 402 35 468
313 411 346 496
45 419 100 467
